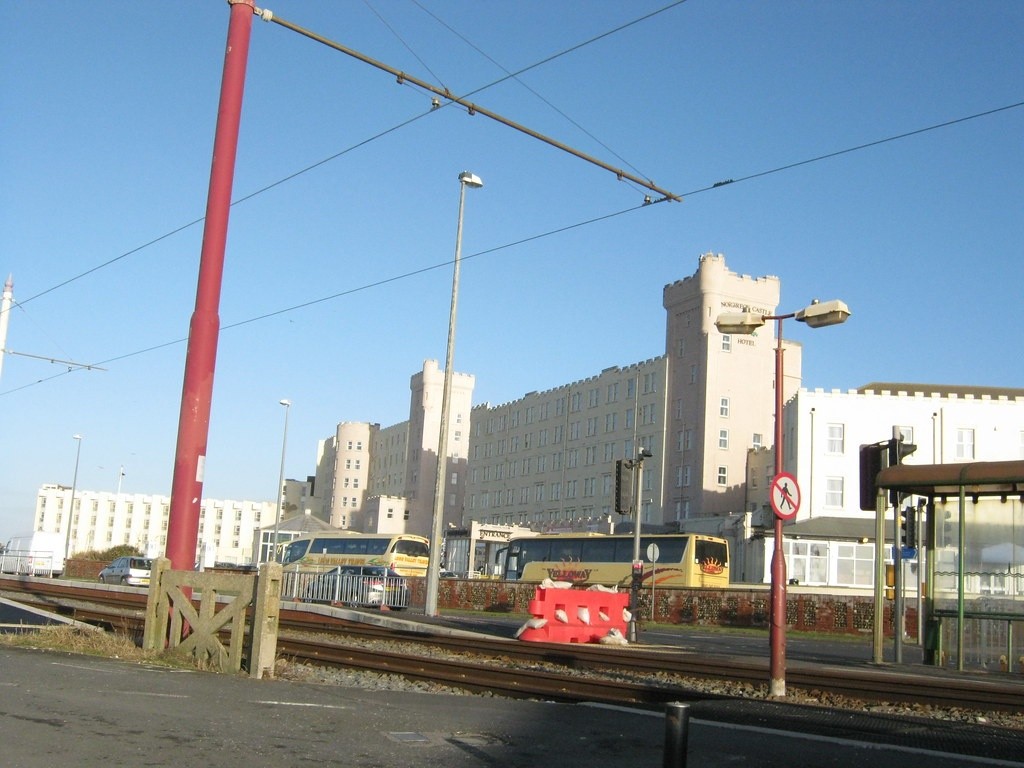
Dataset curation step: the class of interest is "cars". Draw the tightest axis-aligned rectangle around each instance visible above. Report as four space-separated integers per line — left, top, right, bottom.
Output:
438 571 459 579
305 565 408 610
97 555 154 588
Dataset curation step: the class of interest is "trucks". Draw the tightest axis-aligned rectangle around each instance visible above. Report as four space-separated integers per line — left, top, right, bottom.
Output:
0 531 71 577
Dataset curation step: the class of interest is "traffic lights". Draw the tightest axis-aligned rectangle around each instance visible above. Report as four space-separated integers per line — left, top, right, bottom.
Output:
935 507 952 548
901 505 916 548
888 436 918 507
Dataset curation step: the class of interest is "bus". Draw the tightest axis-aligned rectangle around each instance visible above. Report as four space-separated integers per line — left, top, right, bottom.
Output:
277 529 430 611
494 531 730 601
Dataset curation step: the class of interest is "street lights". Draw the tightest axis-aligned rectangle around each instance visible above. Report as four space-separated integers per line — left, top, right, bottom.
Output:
423 170 484 610
60 435 83 577
271 399 292 563
713 299 854 702
